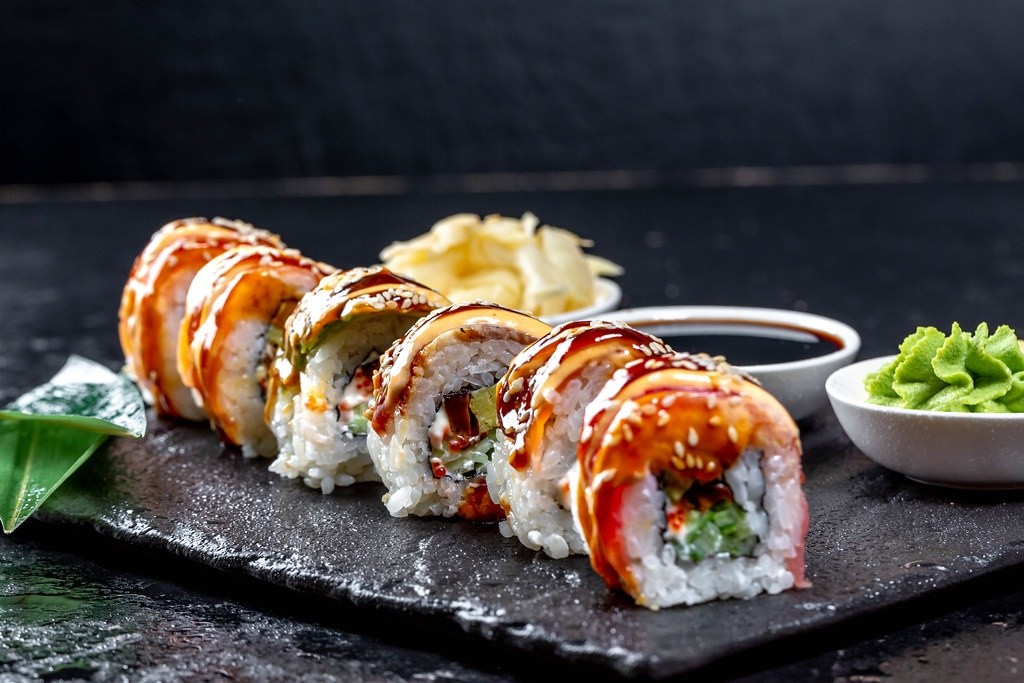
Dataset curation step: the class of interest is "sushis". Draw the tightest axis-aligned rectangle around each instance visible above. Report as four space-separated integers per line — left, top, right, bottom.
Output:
116 215 812 609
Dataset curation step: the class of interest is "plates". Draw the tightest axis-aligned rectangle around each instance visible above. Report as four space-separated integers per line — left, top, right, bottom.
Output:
0 294 1023 683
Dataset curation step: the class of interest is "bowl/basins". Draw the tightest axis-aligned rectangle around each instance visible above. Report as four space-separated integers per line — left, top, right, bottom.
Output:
586 305 865 426
826 356 1023 487
